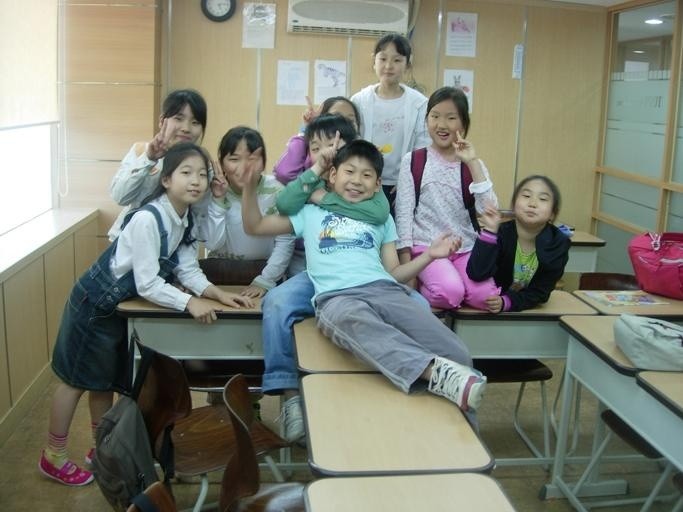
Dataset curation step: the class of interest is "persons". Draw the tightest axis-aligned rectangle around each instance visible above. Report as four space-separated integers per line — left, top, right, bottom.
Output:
272 96 360 186
261 115 430 446
107 88 214 242
205 126 298 421
349 34 433 217
465 175 572 380
238 140 488 438
39 142 255 487
395 87 501 312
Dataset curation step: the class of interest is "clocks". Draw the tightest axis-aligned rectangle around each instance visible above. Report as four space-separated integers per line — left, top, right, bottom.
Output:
200 0 236 22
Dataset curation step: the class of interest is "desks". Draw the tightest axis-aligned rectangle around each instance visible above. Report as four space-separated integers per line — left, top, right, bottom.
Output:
553 227 608 273
115 285 682 510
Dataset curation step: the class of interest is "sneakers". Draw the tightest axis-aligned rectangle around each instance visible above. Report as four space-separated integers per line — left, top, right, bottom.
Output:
426 355 488 413
38 449 95 485
279 396 305 444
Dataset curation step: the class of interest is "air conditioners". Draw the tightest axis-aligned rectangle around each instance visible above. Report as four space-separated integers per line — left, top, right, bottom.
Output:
285 0 409 39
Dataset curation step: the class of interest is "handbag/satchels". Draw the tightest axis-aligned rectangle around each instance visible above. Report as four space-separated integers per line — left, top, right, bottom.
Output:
613 311 682 374
627 230 683 300
